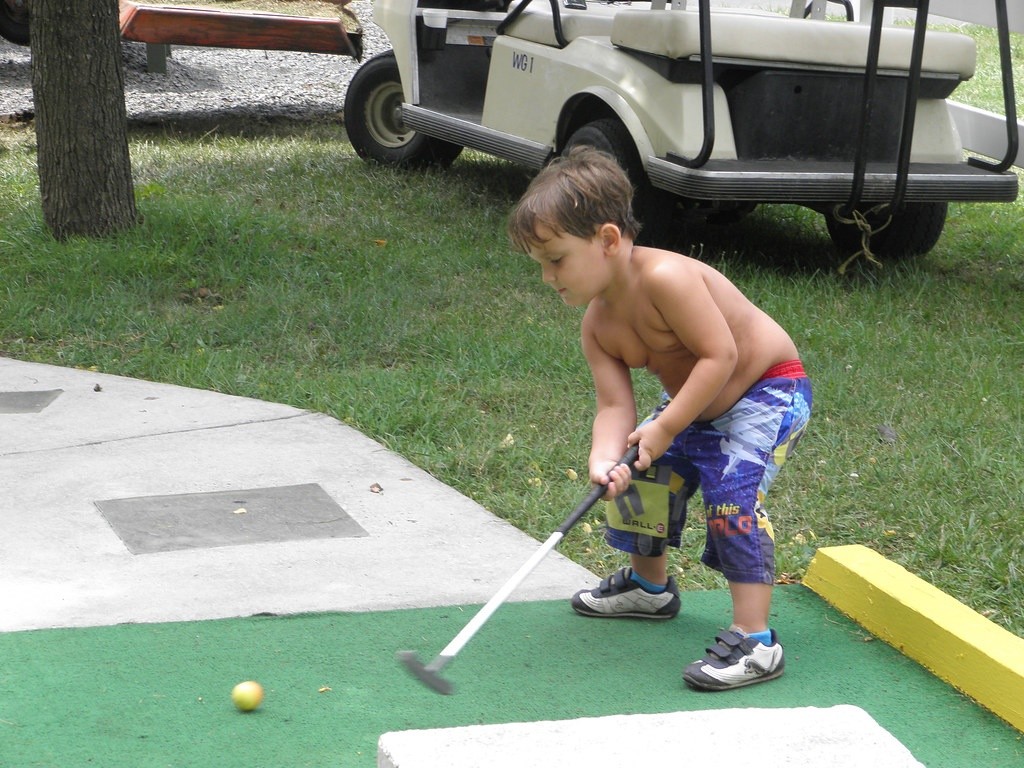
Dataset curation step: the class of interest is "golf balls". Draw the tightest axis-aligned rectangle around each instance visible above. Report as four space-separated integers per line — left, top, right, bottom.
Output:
233 681 262 711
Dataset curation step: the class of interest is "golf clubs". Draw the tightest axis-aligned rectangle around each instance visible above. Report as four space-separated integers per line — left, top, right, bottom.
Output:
396 442 639 696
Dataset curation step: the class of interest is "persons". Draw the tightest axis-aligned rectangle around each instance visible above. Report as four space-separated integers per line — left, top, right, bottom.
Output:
509 148 813 691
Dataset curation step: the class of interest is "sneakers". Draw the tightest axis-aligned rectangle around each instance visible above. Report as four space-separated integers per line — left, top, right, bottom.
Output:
681 625 787 690
570 566 680 619
